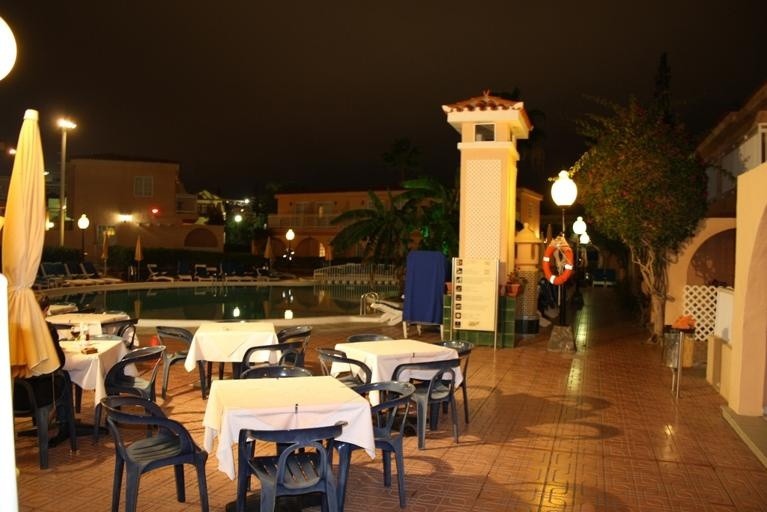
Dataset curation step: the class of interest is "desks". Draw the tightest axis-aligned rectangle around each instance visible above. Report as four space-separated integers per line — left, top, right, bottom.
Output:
200 373 379 510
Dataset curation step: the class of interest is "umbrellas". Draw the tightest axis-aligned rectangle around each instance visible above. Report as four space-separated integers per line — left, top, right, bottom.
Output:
1 108 61 379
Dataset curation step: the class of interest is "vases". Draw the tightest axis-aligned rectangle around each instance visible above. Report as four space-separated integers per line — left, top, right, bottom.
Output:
506 283 524 296
445 282 452 294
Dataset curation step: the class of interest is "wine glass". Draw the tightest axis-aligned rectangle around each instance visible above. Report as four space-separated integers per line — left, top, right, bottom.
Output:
70 326 81 341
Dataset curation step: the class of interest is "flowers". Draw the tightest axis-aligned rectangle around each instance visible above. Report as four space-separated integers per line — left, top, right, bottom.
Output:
506 265 525 287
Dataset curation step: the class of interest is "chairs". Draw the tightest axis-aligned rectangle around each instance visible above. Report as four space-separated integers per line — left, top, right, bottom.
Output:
237 362 318 490
388 356 463 451
97 393 211 511
14 303 472 468
232 419 349 510
323 379 418 508
33 258 295 290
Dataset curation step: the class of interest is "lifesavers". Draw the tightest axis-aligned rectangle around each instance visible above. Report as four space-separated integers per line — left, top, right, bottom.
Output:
543 240 574 285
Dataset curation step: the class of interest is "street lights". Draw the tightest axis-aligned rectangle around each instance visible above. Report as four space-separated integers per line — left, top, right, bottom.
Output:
283 226 295 273
548 167 580 352
75 212 91 262
571 215 588 304
284 287 293 320
55 116 78 248
579 232 591 272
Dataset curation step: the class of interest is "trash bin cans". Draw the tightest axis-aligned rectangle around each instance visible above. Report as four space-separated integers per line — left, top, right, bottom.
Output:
662 325 696 368
